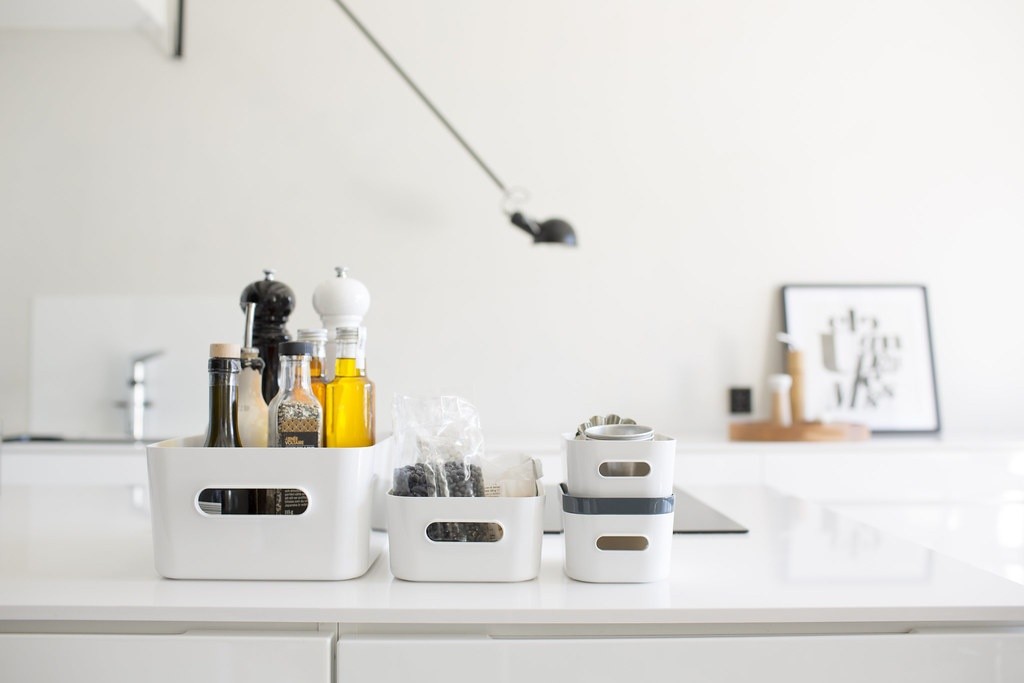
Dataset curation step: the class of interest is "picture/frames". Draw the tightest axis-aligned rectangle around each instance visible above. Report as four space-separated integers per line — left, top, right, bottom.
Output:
779 283 939 432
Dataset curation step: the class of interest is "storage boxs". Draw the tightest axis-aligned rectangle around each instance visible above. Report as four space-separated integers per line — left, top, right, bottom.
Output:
558 482 676 584
146 427 395 582
562 434 677 499
385 482 547 583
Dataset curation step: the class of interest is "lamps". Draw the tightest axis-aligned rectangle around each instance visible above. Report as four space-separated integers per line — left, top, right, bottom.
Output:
510 210 579 248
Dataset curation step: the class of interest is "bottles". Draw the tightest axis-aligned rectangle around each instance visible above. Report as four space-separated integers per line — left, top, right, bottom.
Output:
296 327 329 447
201 357 242 447
268 341 323 447
326 324 376 448
237 357 269 448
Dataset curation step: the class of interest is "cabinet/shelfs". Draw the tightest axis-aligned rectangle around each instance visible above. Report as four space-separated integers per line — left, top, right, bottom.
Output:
0 486 1024 683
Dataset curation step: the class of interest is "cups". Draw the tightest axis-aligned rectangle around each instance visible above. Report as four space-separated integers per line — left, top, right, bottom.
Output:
583 422 654 476
786 348 820 422
